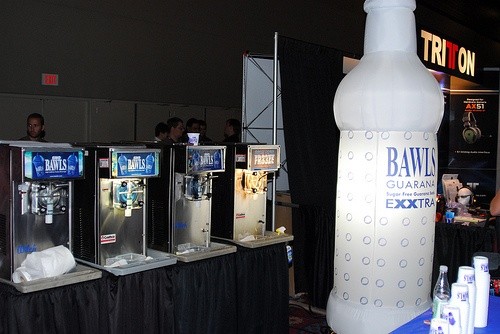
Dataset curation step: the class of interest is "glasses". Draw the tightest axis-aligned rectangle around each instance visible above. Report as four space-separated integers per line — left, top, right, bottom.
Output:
456 195 472 198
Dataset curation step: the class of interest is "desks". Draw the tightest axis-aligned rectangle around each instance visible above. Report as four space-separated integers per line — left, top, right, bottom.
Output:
387 294 500 334
0 241 290 334
430 221 500 296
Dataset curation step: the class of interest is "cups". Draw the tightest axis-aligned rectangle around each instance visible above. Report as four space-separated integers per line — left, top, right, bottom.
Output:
430 256 491 334
446 212 454 223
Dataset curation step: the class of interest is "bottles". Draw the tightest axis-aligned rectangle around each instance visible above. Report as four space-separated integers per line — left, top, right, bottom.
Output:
436 194 445 213
326 0 443 334
432 265 450 312
257 219 265 238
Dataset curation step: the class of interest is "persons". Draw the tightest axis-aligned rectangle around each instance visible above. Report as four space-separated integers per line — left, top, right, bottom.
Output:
197 120 215 144
154 122 168 141
219 118 240 143
167 117 184 147
456 187 472 207
185 118 205 146
17 112 48 143
